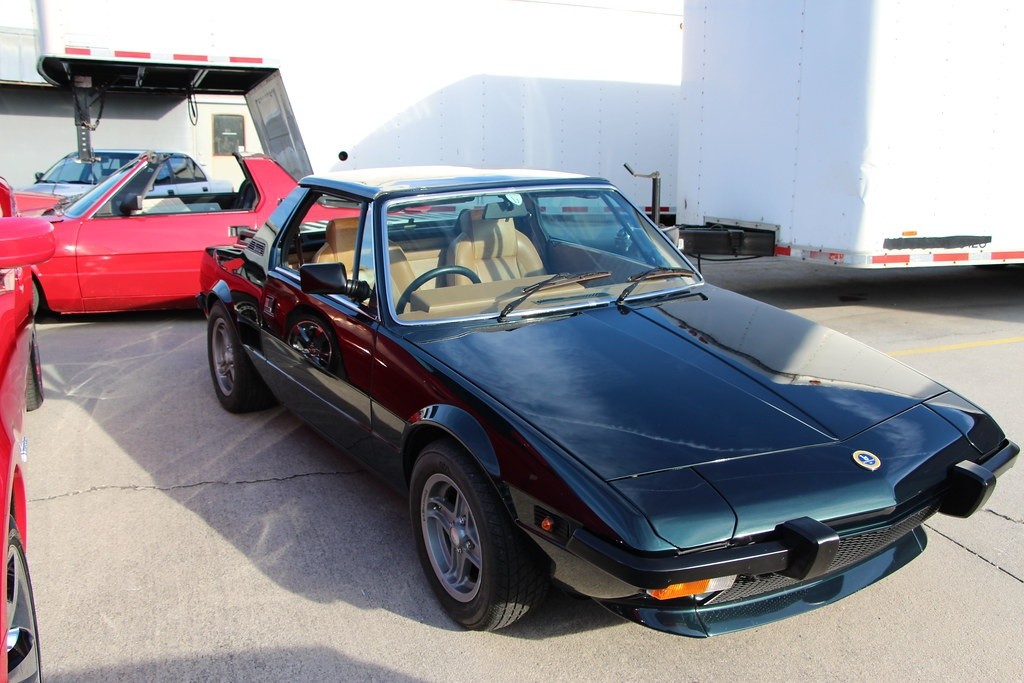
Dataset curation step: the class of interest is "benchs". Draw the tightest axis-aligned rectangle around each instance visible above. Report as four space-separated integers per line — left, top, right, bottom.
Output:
278 223 463 287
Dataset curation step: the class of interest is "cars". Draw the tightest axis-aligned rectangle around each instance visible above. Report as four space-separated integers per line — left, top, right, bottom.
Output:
1 176 57 683
12 150 236 214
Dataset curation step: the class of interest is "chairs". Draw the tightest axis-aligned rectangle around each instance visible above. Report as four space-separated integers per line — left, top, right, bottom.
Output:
449 209 548 287
316 219 426 318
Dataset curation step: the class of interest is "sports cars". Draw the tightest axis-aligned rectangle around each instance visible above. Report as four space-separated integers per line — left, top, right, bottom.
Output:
196 163 1022 641
18 151 432 322
